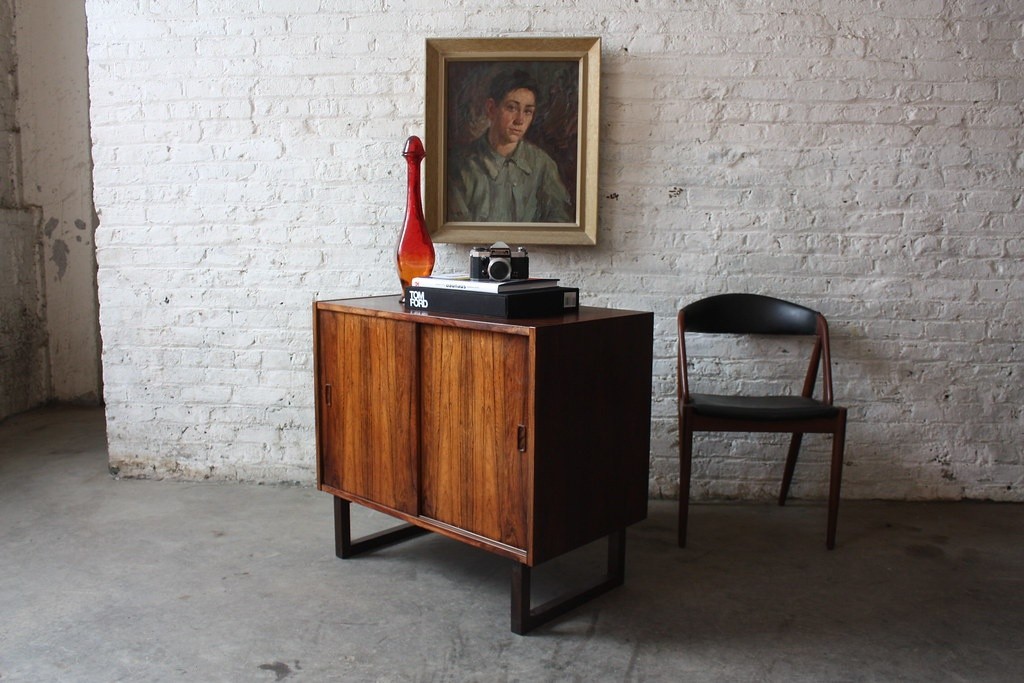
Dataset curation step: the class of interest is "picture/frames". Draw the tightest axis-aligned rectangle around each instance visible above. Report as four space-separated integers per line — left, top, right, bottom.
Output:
427 34 603 244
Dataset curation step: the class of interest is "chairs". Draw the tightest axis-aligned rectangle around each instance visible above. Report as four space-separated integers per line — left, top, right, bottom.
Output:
677 292 849 553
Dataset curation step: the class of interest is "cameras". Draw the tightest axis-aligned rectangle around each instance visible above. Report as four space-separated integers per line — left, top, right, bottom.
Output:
470 241 529 282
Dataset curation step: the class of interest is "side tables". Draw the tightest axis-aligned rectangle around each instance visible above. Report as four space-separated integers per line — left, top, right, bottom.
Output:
312 292 654 636
